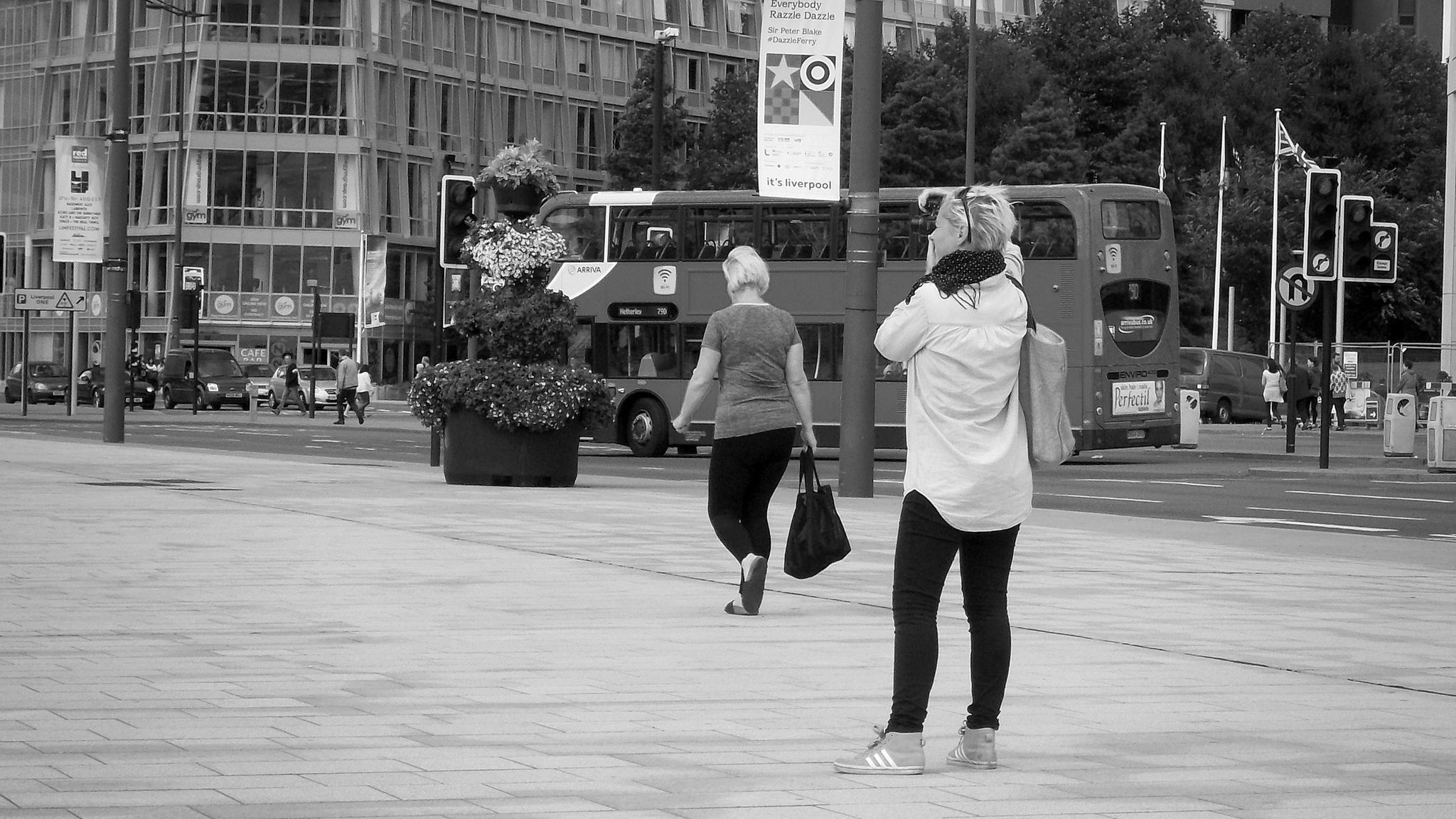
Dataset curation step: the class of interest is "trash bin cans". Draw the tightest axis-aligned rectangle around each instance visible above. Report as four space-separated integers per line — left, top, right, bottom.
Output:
1383 392 1416 456
1427 396 1456 474
1175 389 1201 449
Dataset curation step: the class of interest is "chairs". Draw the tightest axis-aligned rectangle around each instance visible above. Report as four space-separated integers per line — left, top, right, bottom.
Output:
620 228 1078 257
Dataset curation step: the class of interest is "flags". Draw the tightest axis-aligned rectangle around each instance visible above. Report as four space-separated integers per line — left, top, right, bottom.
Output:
1224 124 1243 183
1276 118 1320 169
1163 129 1180 184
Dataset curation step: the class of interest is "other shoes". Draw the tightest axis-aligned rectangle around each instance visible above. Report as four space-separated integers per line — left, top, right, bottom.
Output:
334 420 344 424
300 411 306 416
359 417 364 424
271 408 279 415
740 556 769 613
1337 424 1348 431
724 600 760 616
1280 421 1285 429
1264 428 1272 430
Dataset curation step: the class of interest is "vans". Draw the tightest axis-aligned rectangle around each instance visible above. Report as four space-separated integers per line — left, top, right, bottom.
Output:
1180 346 1288 425
161 349 250 411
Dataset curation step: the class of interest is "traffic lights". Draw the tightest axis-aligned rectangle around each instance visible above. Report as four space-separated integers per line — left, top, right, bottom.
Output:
179 290 197 329
440 176 480 269
1304 163 1399 283
126 290 141 329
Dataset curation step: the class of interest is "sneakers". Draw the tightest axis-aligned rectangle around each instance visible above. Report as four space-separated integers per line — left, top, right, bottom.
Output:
832 729 926 773
945 727 999 770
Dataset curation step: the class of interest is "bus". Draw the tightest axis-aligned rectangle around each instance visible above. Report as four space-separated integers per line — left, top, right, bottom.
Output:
538 183 1181 459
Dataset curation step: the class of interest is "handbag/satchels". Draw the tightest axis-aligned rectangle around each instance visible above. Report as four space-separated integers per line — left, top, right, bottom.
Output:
784 447 851 580
1278 369 1288 394
1004 272 1075 472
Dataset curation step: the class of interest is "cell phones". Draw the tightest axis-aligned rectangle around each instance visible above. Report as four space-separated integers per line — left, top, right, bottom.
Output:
924 194 944 211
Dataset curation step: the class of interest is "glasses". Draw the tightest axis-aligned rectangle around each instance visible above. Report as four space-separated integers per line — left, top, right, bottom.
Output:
954 185 973 244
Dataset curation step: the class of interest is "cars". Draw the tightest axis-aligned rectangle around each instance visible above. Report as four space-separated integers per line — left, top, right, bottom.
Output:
77 367 155 411
268 365 349 411
4 360 68 405
237 362 276 404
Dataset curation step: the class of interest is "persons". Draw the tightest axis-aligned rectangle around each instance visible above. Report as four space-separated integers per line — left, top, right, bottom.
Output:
1393 361 1423 432
1306 357 1320 429
835 187 1035 774
1375 377 1390 402
343 362 361 418
1329 364 1347 431
1287 356 1310 431
271 354 307 417
126 354 165 397
356 364 374 418
1329 353 1348 429
672 246 817 615
1262 358 1285 430
332 349 364 425
